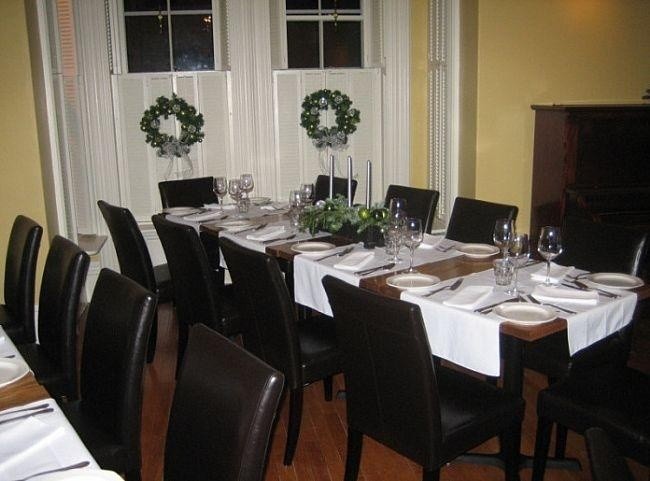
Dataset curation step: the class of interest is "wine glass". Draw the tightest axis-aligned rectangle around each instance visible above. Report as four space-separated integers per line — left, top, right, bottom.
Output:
212 173 317 228
539 227 563 290
384 198 423 275
494 219 517 268
507 232 532 296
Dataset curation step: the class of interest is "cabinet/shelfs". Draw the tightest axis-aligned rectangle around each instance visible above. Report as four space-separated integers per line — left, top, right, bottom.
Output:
520 104 650 463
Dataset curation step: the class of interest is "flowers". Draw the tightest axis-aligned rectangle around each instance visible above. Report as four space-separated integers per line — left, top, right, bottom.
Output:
140 93 204 157
300 89 361 147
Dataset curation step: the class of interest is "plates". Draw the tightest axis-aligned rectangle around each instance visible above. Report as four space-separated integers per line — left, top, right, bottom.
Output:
383 272 441 290
162 197 314 244
0 357 32 389
585 273 644 292
454 242 501 263
492 302 560 326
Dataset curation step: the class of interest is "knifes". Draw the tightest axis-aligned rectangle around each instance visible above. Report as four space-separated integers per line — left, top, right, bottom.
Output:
354 262 396 277
316 246 356 263
474 297 523 316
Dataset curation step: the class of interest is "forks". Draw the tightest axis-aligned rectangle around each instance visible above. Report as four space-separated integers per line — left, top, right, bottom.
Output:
422 277 464 297
526 293 578 315
562 283 621 299
